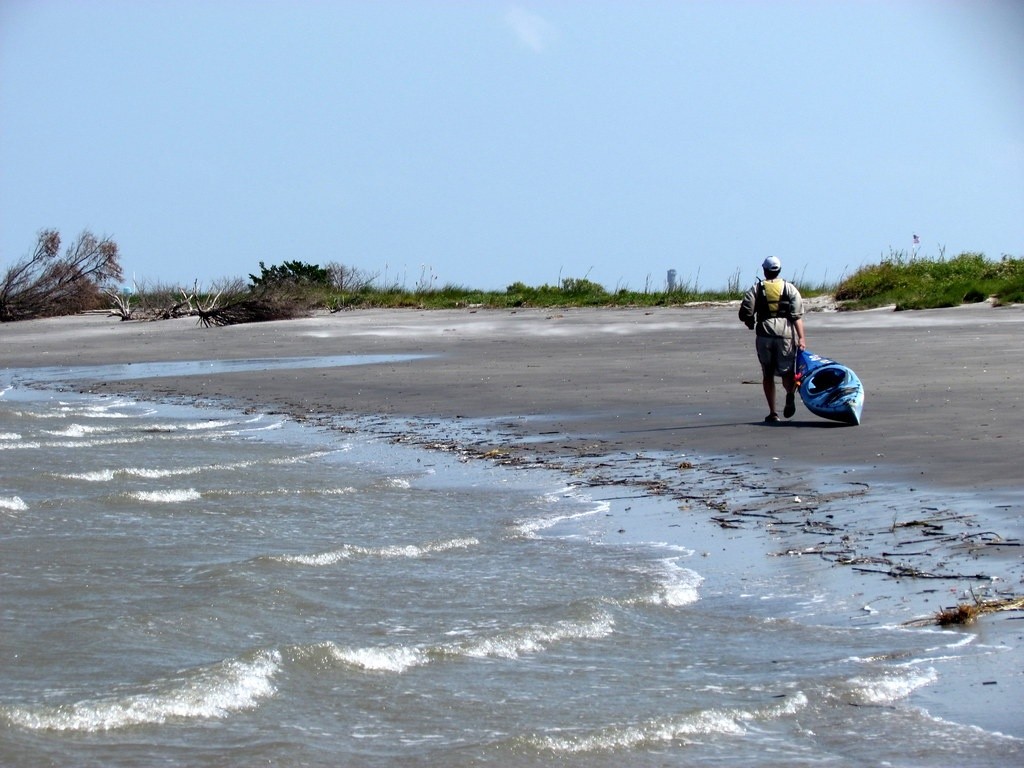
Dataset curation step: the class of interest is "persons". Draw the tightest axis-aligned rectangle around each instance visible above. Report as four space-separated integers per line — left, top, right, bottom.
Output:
738 256 806 421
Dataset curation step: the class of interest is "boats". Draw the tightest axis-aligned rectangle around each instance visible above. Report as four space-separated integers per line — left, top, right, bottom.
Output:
955 297 998 309
794 344 871 424
843 304 899 313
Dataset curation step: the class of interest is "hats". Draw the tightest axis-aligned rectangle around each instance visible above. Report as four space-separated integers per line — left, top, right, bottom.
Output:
762 255 781 272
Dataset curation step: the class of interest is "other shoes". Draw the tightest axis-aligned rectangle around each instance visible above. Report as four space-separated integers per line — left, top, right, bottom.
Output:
784 392 795 418
764 413 781 421
